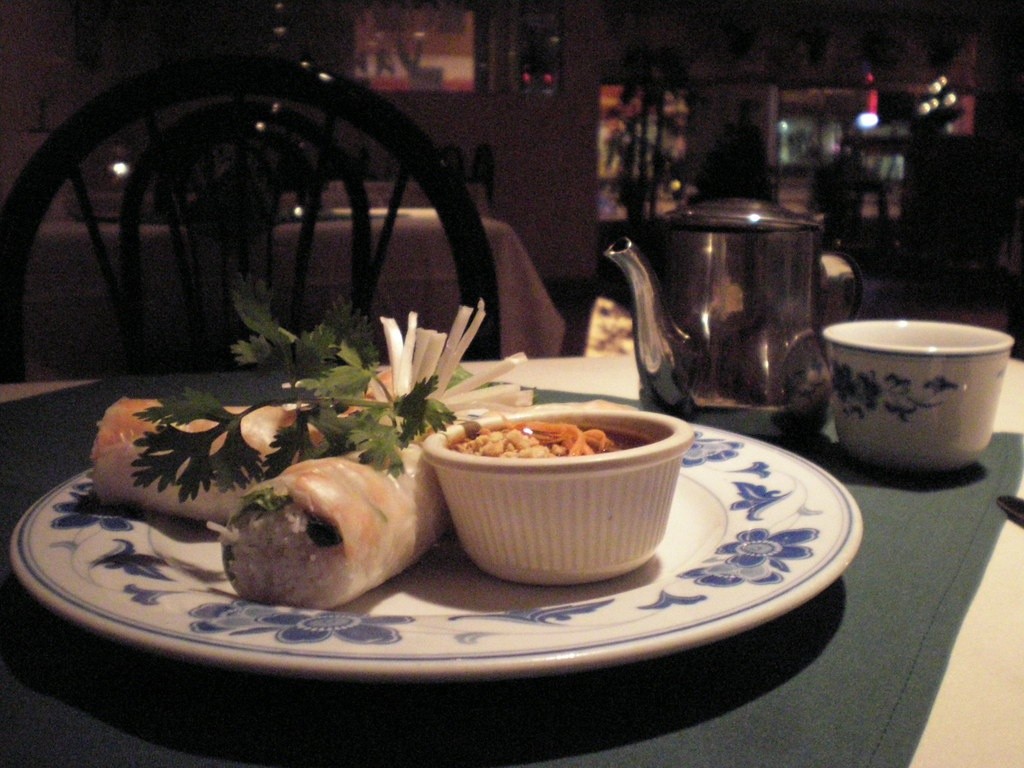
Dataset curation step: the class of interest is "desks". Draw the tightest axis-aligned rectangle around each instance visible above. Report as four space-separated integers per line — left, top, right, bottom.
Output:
0 349 1024 768
0 178 568 385
840 131 983 281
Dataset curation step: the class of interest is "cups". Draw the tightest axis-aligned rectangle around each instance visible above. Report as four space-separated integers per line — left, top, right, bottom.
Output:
822 319 1015 472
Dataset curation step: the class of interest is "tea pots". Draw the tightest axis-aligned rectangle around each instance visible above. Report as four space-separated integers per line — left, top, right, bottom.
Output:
602 162 866 449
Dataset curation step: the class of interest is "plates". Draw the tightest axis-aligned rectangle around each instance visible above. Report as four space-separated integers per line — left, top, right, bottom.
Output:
8 423 865 686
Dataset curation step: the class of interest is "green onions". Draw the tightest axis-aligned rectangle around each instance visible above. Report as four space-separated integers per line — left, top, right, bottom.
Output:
380 299 534 413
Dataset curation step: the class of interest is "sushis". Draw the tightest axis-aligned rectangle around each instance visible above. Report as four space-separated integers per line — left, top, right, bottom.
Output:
93 395 446 606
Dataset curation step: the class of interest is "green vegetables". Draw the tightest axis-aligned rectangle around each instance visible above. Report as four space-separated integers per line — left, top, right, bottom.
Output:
131 291 495 502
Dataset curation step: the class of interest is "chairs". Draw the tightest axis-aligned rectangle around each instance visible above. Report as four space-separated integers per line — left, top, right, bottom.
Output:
840 142 898 223
0 51 510 383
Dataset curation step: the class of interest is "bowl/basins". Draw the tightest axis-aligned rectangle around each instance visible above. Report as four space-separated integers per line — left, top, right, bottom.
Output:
421 406 696 586
